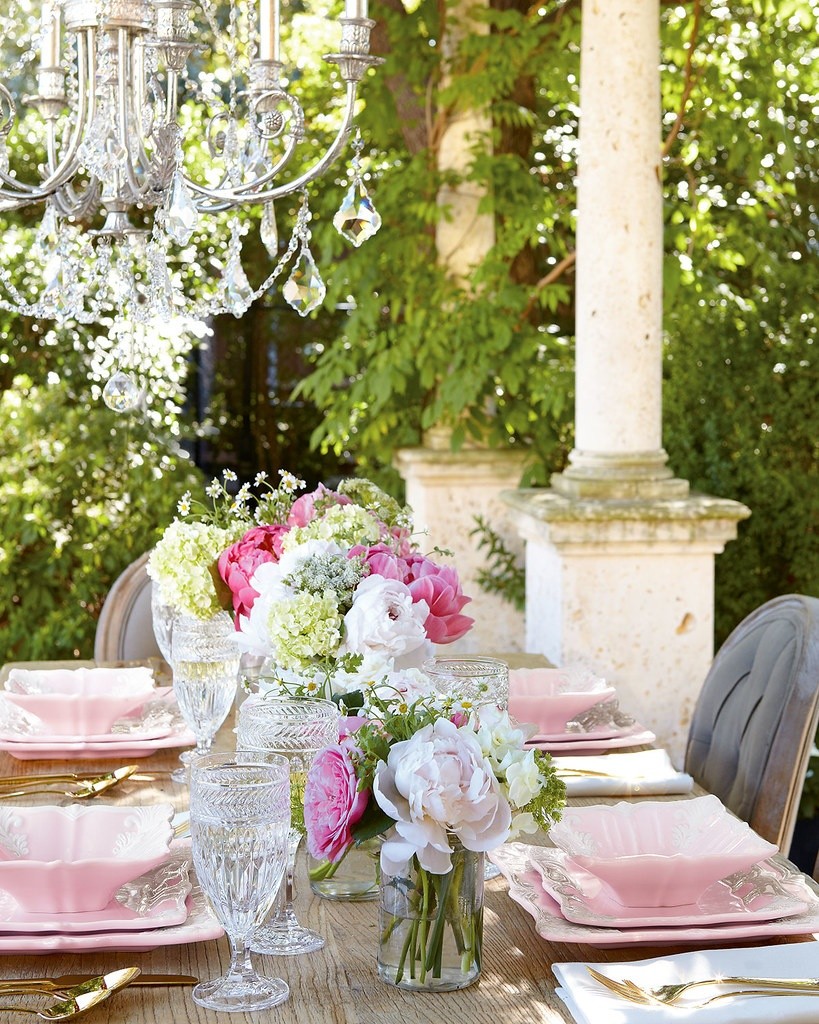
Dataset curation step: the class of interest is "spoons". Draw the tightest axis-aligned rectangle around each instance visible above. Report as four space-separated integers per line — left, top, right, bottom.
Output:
0 990 112 1020
0 967 142 1001
0 775 117 799
0 764 140 793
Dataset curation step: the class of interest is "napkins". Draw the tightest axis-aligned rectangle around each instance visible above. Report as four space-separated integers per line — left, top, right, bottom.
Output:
538 747 694 796
552 941 819 1024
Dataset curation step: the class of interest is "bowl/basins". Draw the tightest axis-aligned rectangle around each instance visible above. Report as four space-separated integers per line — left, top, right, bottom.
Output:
0 805 175 913
509 668 616 728
4 667 155 733
543 795 780 905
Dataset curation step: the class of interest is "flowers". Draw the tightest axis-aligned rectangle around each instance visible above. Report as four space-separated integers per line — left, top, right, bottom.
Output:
149 467 574 982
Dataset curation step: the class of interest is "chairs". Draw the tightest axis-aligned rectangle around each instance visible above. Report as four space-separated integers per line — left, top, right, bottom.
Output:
688 593 819 858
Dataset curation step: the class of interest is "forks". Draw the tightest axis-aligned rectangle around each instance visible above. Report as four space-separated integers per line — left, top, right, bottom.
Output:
621 978 819 1008
587 966 819 1004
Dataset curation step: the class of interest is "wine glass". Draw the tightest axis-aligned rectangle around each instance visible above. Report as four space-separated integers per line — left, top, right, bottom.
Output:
191 753 292 1013
238 697 340 955
171 622 240 782
151 582 226 762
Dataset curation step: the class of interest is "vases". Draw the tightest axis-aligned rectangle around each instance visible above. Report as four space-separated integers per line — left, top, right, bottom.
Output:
373 831 484 996
260 657 390 897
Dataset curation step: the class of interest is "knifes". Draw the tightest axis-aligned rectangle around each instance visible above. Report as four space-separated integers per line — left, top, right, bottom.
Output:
0 974 199 987
0 771 174 781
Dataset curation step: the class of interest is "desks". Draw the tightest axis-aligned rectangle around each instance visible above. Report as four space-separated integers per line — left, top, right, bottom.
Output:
0 655 819 1024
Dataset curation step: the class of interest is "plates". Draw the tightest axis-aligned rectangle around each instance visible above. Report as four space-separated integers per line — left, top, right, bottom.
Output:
0 839 190 932
488 843 819 949
0 708 173 742
513 715 636 741
526 852 807 926
0 837 225 954
0 687 196 760
519 730 656 756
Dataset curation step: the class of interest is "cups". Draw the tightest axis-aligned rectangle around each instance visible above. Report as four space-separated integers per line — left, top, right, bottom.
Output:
423 654 509 718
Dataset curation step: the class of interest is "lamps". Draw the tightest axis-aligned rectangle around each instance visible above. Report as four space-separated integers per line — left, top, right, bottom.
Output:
0 0 383 406
